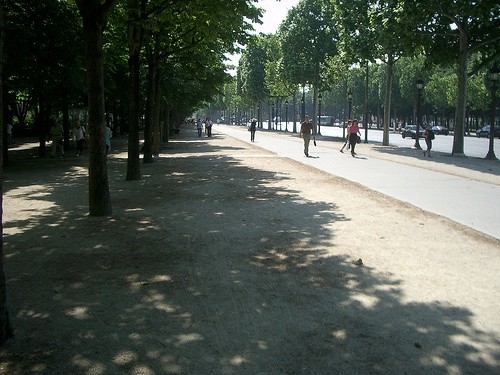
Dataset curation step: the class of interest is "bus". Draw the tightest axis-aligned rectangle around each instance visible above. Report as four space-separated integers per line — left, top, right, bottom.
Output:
316 116 335 125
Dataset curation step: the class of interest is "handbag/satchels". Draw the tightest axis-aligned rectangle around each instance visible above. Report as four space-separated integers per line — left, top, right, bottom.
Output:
346 140 350 150
313 137 316 146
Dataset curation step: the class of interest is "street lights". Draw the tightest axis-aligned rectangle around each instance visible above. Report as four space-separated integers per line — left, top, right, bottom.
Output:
300 97 305 123
413 77 424 147
251 107 253 120
257 105 260 128
484 61 499 161
284 99 289 132
271 103 274 130
347 89 354 120
316 93 323 136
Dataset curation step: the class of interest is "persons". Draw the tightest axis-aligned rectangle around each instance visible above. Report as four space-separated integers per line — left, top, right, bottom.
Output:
104 119 111 159
206 118 212 138
67 120 88 157
205 118 209 134
217 118 219 125
299 115 315 157
347 120 361 158
340 120 356 155
423 124 434 158
250 118 256 142
47 119 65 159
6 122 15 144
197 119 203 138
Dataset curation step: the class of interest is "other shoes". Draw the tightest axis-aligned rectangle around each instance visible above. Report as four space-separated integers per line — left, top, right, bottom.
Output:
304 149 308 157
422 150 432 158
351 152 356 157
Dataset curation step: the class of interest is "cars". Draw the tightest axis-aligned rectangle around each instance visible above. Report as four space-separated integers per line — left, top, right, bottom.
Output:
475 125 500 139
430 125 449 135
340 121 348 128
402 125 426 139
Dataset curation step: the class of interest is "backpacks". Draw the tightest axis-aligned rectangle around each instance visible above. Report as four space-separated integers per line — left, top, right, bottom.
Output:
425 130 435 140
